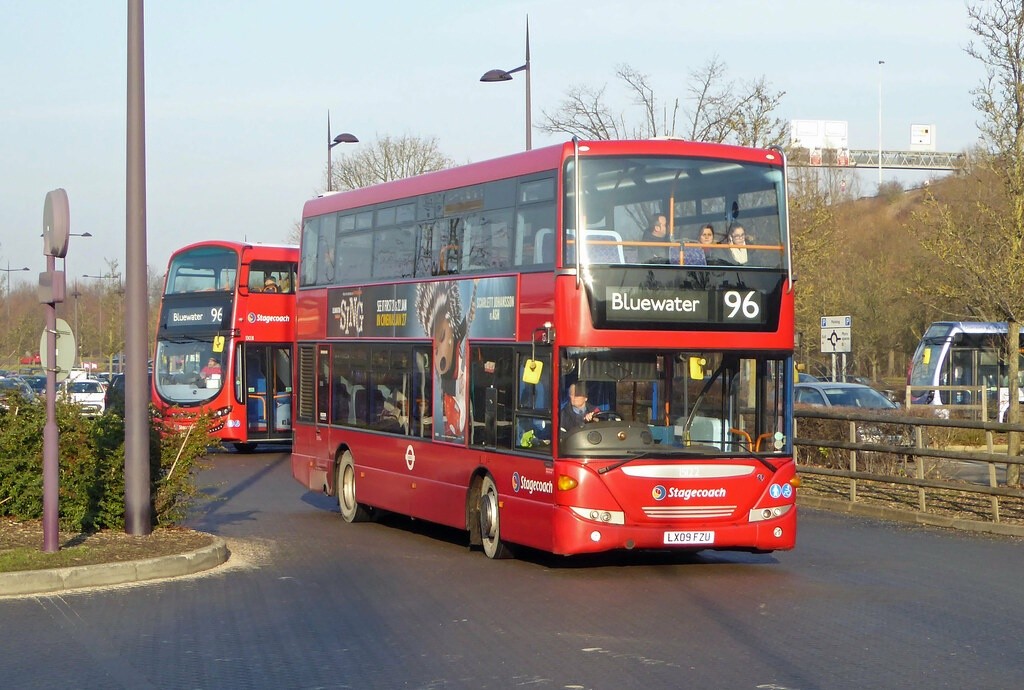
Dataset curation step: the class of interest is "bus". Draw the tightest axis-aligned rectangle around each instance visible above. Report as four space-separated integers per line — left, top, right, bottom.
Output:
150 240 299 452
291 135 804 559
907 322 1024 424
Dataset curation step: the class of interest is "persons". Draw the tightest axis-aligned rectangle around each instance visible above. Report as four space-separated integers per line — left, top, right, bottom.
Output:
953 355 964 379
697 222 756 264
200 358 222 379
638 213 669 264
560 383 600 437
416 397 430 420
265 276 282 293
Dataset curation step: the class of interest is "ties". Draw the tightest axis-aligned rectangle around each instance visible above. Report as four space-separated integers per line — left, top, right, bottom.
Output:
578 410 583 416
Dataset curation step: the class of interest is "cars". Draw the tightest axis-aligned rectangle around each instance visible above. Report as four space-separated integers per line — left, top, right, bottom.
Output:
0 368 123 420
106 355 126 363
21 354 40 365
794 383 899 410
104 375 124 418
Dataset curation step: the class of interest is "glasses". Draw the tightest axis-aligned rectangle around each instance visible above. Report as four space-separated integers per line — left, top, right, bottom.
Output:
733 233 746 240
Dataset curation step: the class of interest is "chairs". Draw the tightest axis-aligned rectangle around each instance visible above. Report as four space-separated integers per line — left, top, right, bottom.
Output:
318 380 431 429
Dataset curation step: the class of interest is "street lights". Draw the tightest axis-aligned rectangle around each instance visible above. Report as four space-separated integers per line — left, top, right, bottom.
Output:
8 267 31 302
479 61 531 152
82 274 122 373
327 132 360 192
41 232 92 367
